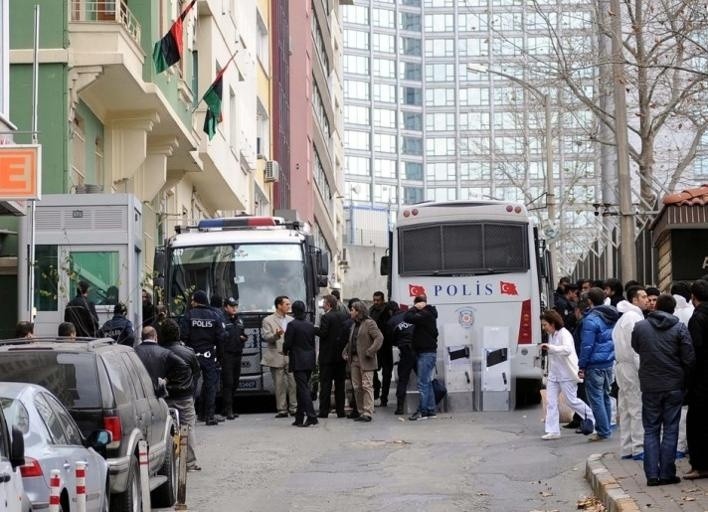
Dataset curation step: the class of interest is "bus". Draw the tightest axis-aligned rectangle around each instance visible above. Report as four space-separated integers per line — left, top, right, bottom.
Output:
375 197 559 409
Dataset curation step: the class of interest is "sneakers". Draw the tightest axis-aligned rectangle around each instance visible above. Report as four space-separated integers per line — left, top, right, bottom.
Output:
622 451 645 462
196 411 239 425
407 409 437 421
348 410 372 423
676 452 687 459
587 433 607 442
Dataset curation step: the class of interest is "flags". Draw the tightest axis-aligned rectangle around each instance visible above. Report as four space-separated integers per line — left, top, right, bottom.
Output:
204 71 224 140
152 17 183 74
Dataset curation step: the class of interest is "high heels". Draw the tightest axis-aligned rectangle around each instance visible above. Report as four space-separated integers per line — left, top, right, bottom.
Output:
292 413 318 428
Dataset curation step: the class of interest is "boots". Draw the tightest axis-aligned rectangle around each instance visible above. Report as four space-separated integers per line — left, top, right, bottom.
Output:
394 397 405 415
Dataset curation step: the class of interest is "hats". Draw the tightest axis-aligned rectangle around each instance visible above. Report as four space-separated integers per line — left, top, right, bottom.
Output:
224 296 239 308
192 290 208 304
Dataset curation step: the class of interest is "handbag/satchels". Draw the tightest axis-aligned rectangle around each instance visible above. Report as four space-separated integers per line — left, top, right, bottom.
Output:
431 362 447 407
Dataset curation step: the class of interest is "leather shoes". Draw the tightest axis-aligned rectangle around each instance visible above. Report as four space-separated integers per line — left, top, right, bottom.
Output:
274 411 289 419
337 414 346 418
645 475 682 487
683 469 707 481
542 432 562 440
319 413 328 418
561 421 582 430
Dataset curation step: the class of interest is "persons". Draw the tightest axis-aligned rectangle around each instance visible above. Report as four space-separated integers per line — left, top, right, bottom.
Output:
534 277 707 487
259 289 438 428
57 281 246 471
14 320 35 338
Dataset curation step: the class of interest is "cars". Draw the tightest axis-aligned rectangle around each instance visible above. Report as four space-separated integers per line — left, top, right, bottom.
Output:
0 400 29 512
0 380 114 512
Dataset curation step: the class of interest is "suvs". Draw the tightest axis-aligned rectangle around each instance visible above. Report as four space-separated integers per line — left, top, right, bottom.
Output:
0 334 181 512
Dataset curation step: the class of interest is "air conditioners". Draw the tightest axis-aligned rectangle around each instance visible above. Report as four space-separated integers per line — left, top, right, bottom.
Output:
265 159 280 184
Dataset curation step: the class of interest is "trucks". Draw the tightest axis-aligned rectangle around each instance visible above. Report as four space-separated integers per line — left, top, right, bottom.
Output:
161 211 329 408
